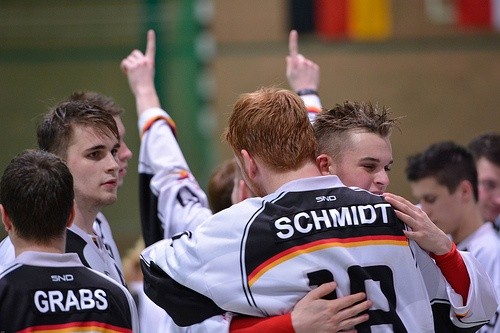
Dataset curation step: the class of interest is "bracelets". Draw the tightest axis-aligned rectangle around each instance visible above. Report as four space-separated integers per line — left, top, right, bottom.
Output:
297 88 319 96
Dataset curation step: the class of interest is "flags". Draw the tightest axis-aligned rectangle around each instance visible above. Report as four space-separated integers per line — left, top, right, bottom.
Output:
424 0 500 26
285 0 392 41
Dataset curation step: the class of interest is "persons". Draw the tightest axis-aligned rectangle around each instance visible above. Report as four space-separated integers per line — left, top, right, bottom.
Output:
207 158 236 215
120 29 323 247
140 87 434 333
405 140 500 333
0 149 139 333
466 134 500 229
52 91 133 269
0 100 128 288
168 101 500 333
